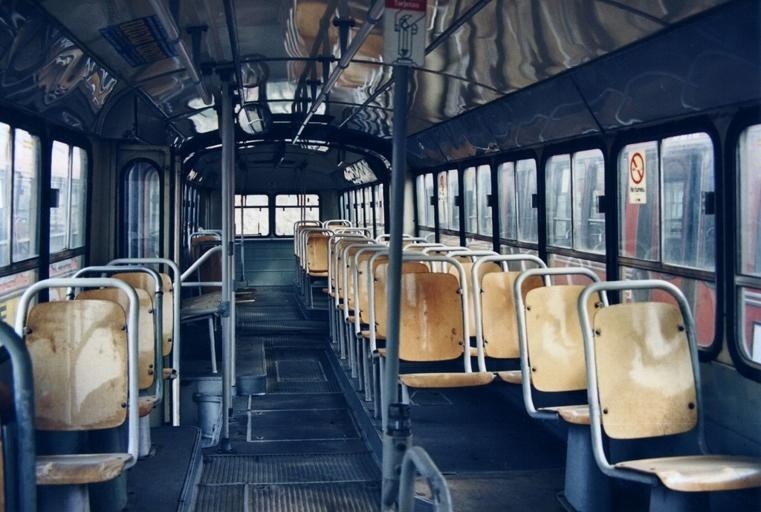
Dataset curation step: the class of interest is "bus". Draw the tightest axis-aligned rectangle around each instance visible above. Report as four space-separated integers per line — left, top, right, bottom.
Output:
425 120 761 362
0 122 83 341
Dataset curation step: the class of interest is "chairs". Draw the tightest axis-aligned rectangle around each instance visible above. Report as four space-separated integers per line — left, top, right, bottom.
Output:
515 265 611 511
66 264 165 456
383 252 498 407
471 251 554 389
292 219 512 408
11 277 142 511
577 277 761 511
99 255 184 430
0 317 43 512
176 225 230 376
